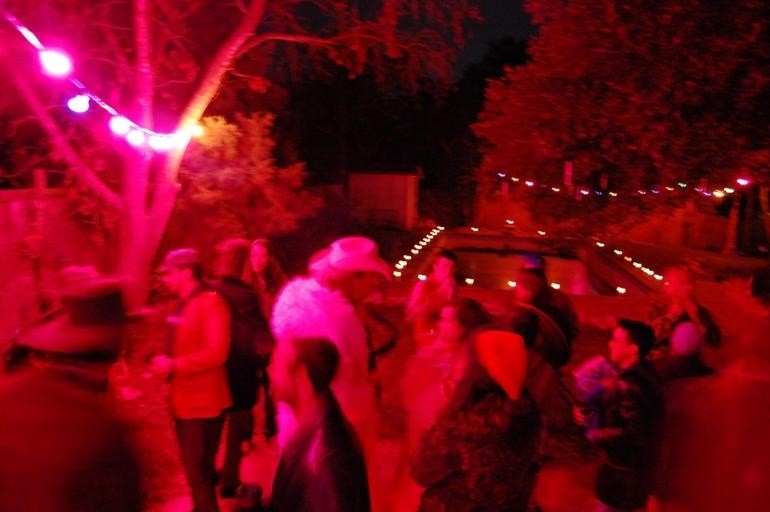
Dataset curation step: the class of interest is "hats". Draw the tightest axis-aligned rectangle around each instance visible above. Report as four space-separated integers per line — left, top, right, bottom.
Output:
670 321 702 361
11 282 150 353
310 236 392 281
471 330 529 399
154 248 202 274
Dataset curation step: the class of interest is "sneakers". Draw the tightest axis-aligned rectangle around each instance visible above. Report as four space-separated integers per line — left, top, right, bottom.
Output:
221 480 260 500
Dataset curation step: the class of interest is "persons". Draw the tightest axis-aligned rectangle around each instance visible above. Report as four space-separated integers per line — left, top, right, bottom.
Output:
0 234 724 512
